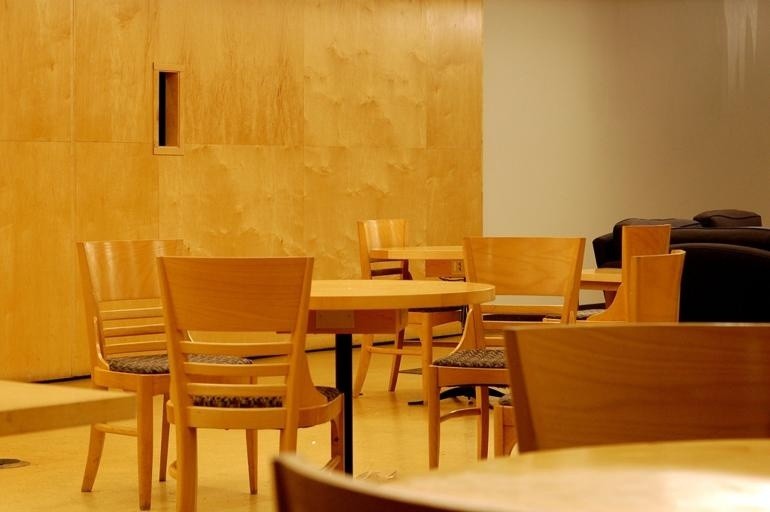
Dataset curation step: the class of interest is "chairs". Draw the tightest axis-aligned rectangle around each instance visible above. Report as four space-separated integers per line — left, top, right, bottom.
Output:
269 452 489 509
494 250 686 458
426 236 588 470
353 219 465 406
578 224 672 319
154 255 346 512
503 320 770 453
592 209 769 323
77 240 259 511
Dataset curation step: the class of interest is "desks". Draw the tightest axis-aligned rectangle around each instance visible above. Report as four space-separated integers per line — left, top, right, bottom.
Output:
387 438 770 512
370 245 505 405
273 278 496 477
